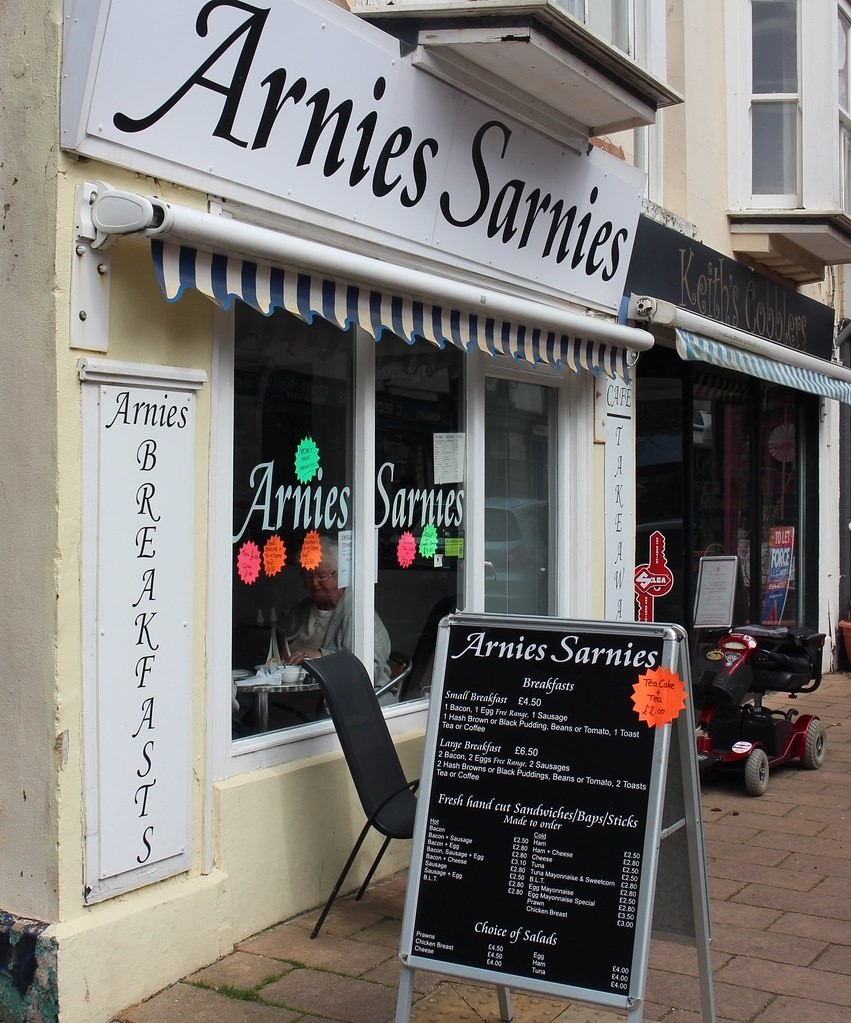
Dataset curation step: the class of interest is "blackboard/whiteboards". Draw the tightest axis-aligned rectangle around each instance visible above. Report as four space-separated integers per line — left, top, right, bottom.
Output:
394 615 684 1008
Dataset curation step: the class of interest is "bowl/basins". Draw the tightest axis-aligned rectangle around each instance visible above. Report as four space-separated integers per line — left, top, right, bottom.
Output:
277 665 308 683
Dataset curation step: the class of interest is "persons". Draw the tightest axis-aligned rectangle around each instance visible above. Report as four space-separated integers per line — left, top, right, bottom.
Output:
271 537 396 715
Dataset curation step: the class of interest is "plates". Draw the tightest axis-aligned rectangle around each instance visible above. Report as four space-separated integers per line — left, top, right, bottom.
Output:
232 670 249 677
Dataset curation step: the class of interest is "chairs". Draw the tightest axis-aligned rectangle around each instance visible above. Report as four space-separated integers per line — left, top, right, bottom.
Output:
374 651 413 706
302 649 421 939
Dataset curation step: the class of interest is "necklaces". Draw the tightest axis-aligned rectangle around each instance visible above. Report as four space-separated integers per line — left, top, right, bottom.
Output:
313 606 333 624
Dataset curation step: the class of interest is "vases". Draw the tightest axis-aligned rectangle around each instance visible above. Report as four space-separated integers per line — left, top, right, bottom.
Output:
839 621 851 664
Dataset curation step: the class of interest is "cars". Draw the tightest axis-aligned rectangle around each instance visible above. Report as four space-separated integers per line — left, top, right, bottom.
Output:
393 499 548 613
371 537 499 690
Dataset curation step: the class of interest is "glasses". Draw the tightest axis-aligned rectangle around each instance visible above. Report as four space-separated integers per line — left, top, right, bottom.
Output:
304 571 336 581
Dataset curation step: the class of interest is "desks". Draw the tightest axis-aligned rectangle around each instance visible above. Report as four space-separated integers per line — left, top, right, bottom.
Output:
231 668 317 734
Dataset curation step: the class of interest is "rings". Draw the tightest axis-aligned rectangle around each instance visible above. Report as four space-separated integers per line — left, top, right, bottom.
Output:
303 654 307 657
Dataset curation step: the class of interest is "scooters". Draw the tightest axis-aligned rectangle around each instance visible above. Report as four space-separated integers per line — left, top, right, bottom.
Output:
690 622 826 798
